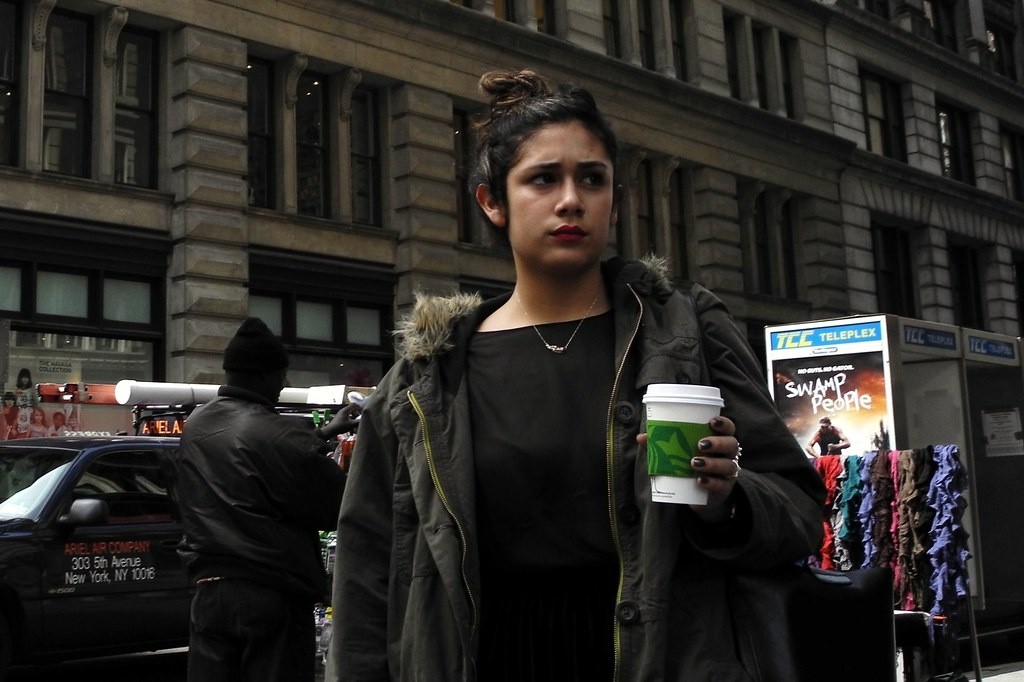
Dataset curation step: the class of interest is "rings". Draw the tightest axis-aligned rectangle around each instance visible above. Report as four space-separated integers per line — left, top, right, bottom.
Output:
735 443 742 459
732 459 741 478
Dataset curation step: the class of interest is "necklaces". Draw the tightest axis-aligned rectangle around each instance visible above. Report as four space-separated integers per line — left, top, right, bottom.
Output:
515 284 604 353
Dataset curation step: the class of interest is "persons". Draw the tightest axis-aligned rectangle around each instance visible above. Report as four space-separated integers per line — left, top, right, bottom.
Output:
48 413 68 436
25 408 48 437
175 317 361 682
806 417 850 458
0 392 19 440
326 73 827 682
15 368 40 438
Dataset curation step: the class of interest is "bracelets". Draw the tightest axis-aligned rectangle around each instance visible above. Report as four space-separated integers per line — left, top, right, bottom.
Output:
729 503 736 521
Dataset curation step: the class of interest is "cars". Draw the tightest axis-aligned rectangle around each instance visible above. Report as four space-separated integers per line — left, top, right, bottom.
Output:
1 434 192 672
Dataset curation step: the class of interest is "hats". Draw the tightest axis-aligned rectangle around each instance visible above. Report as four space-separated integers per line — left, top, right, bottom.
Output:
222 318 289 372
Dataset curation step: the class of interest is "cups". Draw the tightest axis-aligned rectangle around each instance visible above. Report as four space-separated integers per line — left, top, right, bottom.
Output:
641 382 726 505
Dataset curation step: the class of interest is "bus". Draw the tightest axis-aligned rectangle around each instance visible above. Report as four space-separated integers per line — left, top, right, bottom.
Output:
32 372 372 475
